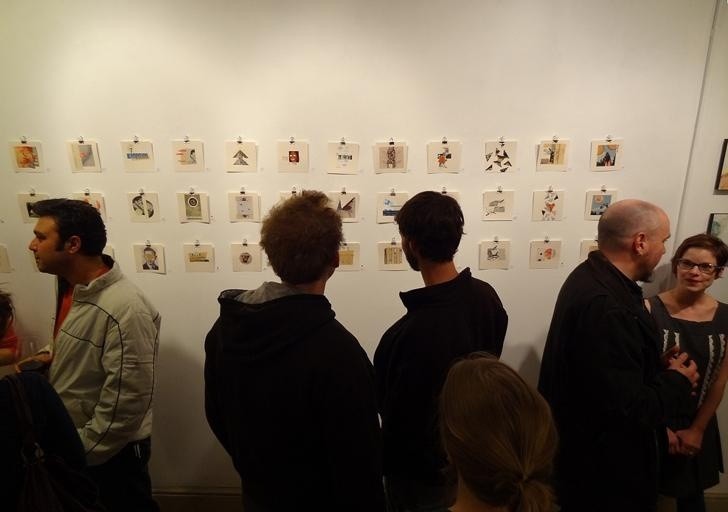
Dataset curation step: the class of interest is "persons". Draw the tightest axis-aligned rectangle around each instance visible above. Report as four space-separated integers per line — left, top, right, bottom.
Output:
0 293 23 364
0 290 86 512
143 246 160 270
645 234 728 511
204 191 382 512
15 198 162 512
438 354 555 512
536 199 699 511
373 191 509 511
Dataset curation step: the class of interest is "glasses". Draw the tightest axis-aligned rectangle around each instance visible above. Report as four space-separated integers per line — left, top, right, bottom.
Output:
675 258 723 274
